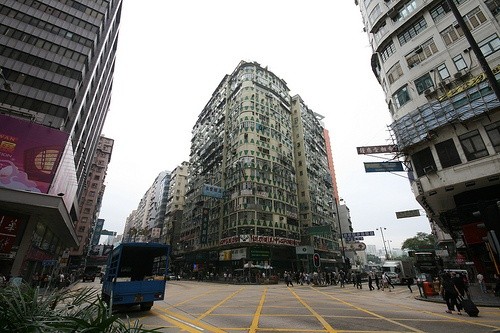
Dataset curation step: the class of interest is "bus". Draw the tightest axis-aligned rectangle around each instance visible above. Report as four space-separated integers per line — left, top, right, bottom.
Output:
86 255 108 276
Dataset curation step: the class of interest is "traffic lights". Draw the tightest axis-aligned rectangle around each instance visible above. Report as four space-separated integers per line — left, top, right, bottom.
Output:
313 253 321 268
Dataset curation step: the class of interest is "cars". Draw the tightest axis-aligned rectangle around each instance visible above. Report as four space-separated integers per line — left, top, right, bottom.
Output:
417 272 433 287
101 264 107 283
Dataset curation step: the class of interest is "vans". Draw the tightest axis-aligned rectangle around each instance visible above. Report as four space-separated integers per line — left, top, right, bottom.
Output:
159 268 176 281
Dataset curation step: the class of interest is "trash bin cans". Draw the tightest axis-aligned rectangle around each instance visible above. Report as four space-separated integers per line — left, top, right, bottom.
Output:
423 281 434 294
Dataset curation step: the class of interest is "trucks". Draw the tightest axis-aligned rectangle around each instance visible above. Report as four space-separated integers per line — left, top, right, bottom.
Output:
381 256 415 286
82 265 98 282
99 242 170 316
405 249 440 276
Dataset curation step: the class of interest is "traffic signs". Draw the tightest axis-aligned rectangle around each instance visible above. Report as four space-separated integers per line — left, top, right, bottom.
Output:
345 241 367 251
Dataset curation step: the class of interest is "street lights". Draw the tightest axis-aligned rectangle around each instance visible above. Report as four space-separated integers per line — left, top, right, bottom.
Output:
375 227 393 260
328 197 345 260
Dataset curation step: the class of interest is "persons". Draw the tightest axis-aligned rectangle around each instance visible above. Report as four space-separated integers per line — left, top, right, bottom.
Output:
178 271 202 281
405 275 412 293
257 272 279 280
312 272 336 287
477 274 487 294
0 272 24 282
32 272 70 289
337 270 351 287
375 275 381 289
381 274 394 292
440 273 467 315
284 271 311 286
206 271 240 281
352 271 362 289
492 274 500 295
368 276 375 291
417 278 426 298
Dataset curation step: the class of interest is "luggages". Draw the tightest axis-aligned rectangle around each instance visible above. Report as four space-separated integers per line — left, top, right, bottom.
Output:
462 298 479 316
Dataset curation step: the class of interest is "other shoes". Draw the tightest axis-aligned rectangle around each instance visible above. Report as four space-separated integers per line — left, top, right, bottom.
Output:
446 310 452 314
457 311 461 315
370 287 374 290
